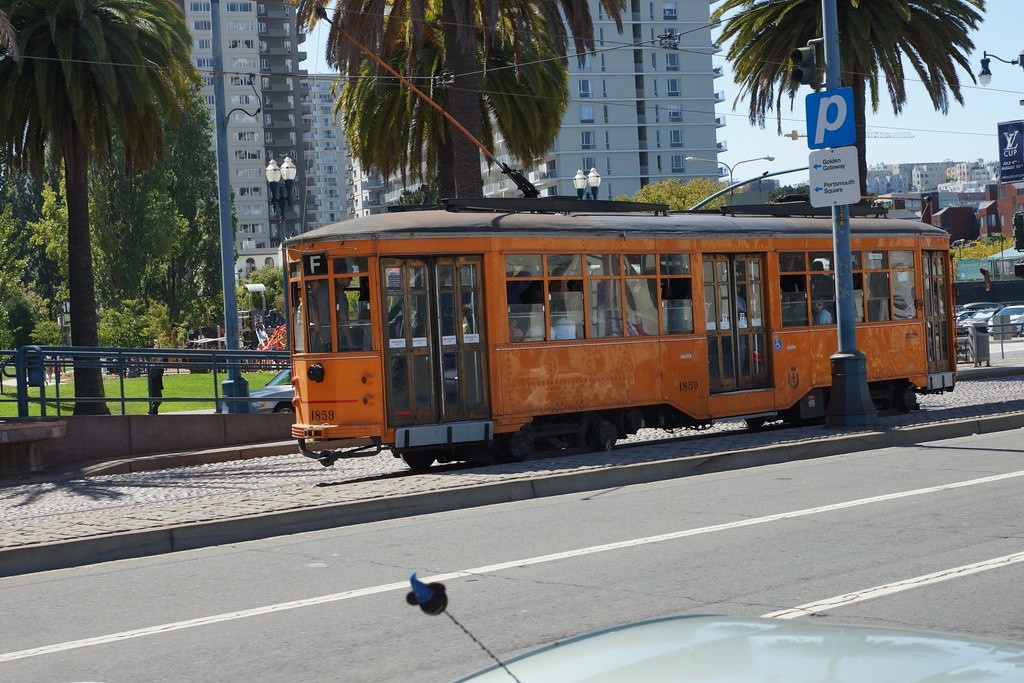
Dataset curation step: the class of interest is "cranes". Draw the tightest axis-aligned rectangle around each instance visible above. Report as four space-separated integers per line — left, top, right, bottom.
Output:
783 130 915 141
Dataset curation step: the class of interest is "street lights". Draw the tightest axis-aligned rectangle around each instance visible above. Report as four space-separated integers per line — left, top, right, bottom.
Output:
682 155 776 207
264 157 296 362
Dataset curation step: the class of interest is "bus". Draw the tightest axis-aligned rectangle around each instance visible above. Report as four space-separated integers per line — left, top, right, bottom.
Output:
281 3 959 473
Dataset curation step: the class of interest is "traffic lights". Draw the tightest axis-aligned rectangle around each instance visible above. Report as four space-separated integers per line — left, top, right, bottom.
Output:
790 45 818 85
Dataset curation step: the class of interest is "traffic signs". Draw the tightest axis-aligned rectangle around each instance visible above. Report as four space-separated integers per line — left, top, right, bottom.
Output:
809 145 861 208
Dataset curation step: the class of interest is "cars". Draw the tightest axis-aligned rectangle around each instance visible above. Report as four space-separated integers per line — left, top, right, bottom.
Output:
218 366 297 417
444 610 1023 683
950 301 1024 337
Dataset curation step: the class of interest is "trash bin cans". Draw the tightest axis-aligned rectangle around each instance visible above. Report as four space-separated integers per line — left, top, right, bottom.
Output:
26 355 46 387
969 322 990 367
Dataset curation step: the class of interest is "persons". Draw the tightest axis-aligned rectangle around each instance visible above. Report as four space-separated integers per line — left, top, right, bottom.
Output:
509 254 580 342
154 339 159 348
311 263 356 352
264 309 285 328
47 357 62 383
780 254 836 325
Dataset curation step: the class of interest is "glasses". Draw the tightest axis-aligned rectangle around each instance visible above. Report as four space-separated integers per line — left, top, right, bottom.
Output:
813 304 822 306
